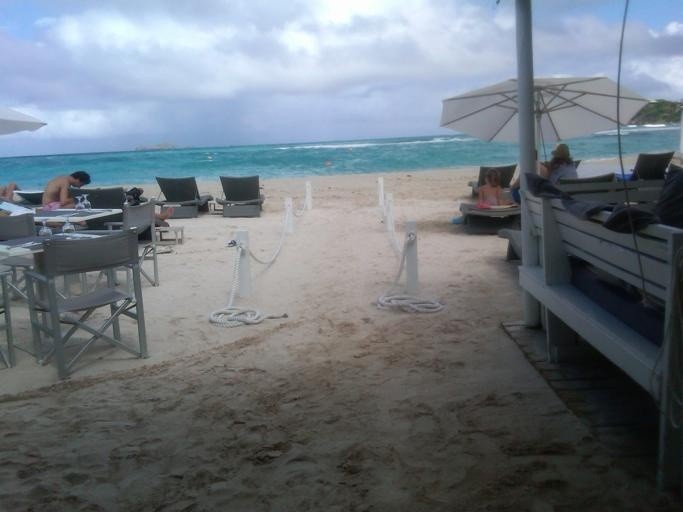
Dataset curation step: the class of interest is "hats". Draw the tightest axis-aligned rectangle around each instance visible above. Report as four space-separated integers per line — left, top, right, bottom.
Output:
550 143 571 161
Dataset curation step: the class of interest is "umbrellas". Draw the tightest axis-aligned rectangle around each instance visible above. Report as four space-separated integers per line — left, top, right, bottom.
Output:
0 107 48 135
440 76 651 174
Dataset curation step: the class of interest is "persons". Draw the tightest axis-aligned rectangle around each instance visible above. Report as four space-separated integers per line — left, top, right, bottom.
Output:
512 150 548 203
41 171 91 208
0 183 22 201
548 144 579 185
477 168 503 206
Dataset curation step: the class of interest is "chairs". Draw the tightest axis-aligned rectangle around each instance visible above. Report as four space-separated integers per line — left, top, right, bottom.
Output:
0 175 158 365
23 226 149 381
215 173 265 218
458 147 676 232
155 174 214 217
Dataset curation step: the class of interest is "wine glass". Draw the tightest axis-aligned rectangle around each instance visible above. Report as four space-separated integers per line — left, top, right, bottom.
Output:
38 218 53 242
74 196 86 216
61 215 76 241
81 194 91 215
